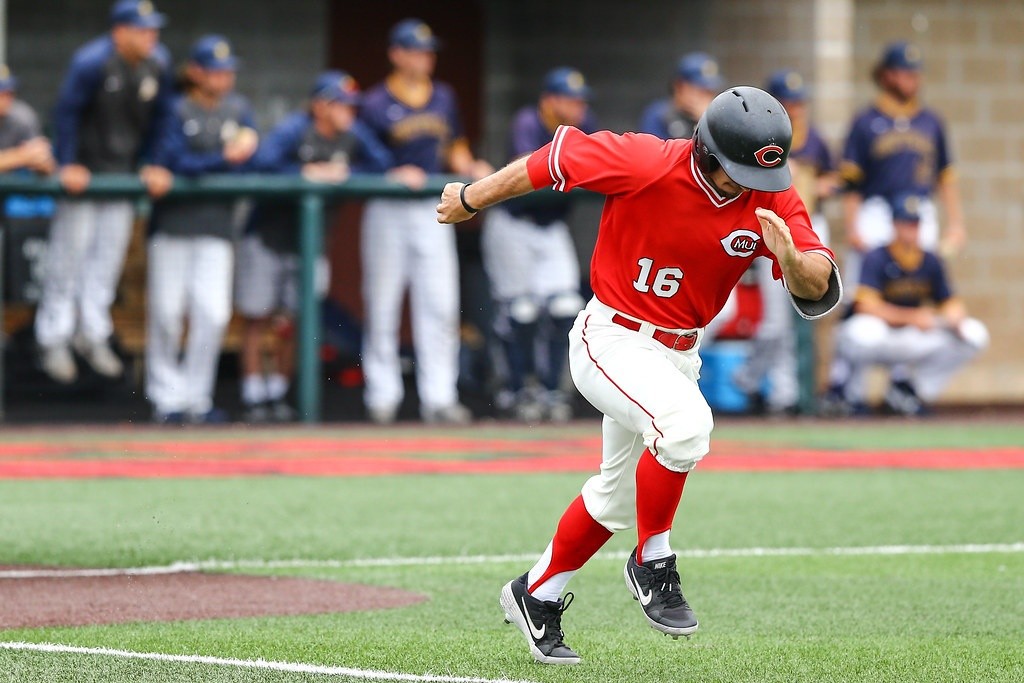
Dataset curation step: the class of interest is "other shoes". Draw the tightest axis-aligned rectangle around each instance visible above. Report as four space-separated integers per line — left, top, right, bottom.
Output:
154 395 214 425
513 382 576 426
883 378 927 416
366 405 398 425
238 376 298 424
824 385 869 418
40 339 122 381
419 402 479 426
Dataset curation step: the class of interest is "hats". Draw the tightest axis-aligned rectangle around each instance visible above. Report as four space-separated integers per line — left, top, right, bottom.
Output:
770 71 809 101
542 69 591 99
666 53 729 91
189 33 240 72
895 198 922 224
312 70 365 106
109 0 168 30
875 44 922 70
390 18 440 51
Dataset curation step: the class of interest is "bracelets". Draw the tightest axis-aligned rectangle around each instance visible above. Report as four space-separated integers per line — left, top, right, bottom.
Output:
459 182 482 215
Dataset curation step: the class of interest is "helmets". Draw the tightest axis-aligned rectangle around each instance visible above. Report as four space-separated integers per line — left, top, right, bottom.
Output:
696 85 796 194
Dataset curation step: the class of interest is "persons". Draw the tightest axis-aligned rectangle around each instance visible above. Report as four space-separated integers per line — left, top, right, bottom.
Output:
483 67 600 420
352 23 497 424
233 71 430 425
34 2 188 382
434 85 836 663
832 195 990 416
0 61 57 352
650 54 845 416
138 35 261 425
821 45 970 416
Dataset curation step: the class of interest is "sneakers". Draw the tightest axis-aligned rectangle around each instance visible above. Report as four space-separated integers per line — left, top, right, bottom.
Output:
498 571 581 667
625 547 699 639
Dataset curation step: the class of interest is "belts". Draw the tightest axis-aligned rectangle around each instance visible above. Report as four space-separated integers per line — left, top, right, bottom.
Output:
612 314 701 351
